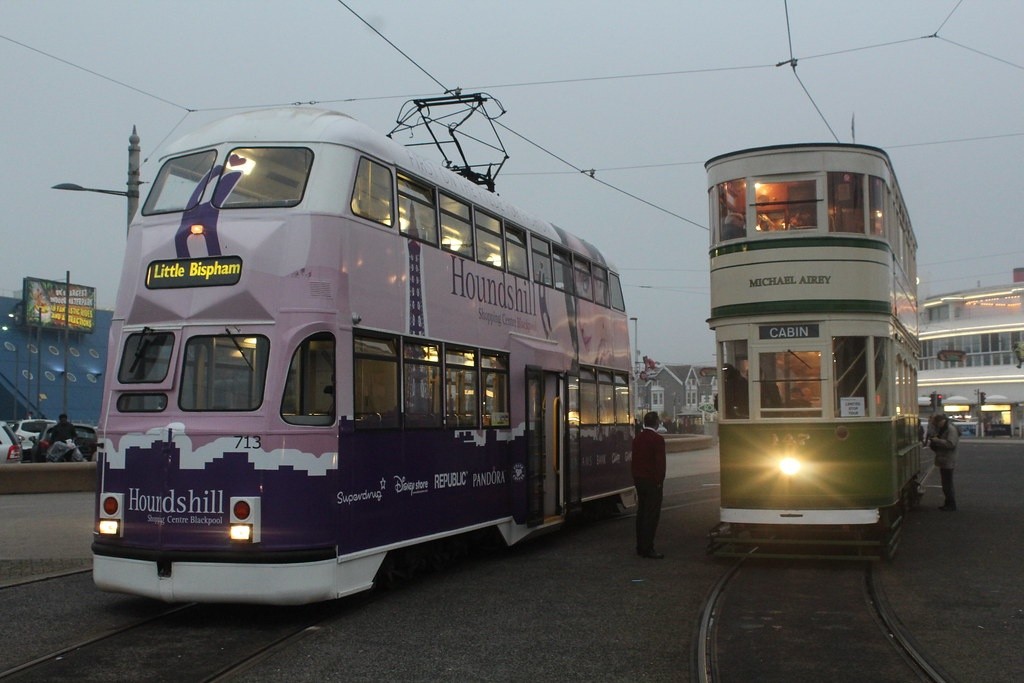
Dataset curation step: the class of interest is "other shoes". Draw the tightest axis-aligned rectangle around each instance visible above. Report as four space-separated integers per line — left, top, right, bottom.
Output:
640 549 665 559
938 501 957 512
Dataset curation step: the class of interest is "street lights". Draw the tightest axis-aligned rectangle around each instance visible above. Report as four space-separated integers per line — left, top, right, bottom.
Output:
1 325 21 420
9 311 32 420
628 316 638 424
49 123 143 239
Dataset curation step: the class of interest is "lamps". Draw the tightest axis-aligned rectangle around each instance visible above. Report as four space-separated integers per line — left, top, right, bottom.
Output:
95 372 102 379
60 371 65 376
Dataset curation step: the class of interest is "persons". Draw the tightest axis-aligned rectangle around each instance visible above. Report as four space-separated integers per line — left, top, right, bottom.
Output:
789 388 812 416
550 223 614 364
918 419 925 445
635 419 641 437
47 414 77 462
715 364 781 419
27 411 36 419
721 211 818 241
929 415 959 512
631 412 666 559
664 416 691 434
923 416 935 447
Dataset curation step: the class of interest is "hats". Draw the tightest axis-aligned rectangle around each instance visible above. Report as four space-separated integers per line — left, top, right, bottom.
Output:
932 415 944 424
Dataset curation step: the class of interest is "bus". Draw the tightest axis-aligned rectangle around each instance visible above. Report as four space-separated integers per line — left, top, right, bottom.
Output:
90 86 637 611
701 140 969 563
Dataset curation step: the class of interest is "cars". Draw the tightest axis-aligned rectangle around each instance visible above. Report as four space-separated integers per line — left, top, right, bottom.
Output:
0 416 100 464
643 423 668 434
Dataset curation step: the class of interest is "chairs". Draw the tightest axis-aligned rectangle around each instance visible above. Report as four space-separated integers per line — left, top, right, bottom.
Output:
352 410 462 428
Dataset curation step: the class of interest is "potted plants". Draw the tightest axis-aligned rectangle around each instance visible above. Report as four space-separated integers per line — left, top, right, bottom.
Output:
1014 426 1020 436
